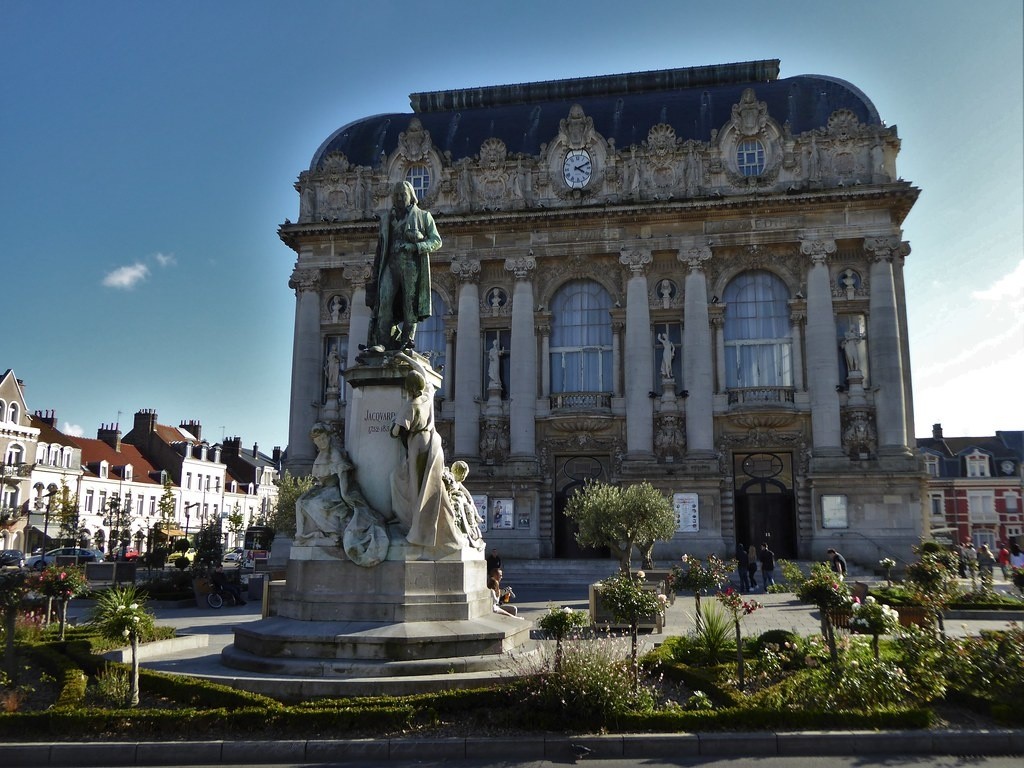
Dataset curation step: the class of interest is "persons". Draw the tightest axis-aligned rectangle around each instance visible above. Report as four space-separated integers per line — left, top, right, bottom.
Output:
758 543 777 591
366 181 443 352
843 269 856 289
325 342 344 388
444 461 486 541
487 340 505 389
295 422 357 547
826 549 847 581
487 568 523 619
843 323 862 370
950 540 1024 589
661 280 672 297
211 565 247 606
735 543 750 595
746 545 759 591
657 333 675 378
332 295 344 315
487 549 502 575
490 288 502 307
391 356 445 537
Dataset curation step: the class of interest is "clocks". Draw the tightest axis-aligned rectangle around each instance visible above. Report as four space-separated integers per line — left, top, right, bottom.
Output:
563 155 592 183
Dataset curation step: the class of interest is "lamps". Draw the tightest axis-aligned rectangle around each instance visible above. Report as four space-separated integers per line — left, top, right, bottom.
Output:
538 304 544 311
661 447 676 463
857 444 871 461
796 290 804 299
648 391 662 399
712 295 718 303
614 300 621 308
676 390 689 398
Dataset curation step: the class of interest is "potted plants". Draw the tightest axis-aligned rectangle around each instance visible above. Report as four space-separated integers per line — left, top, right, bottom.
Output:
570 474 676 634
868 588 924 630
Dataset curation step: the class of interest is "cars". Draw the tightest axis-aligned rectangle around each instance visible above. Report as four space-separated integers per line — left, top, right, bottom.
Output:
25 547 140 572
223 547 244 563
168 548 198 563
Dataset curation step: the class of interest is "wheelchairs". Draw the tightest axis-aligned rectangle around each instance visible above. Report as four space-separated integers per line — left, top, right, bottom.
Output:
208 584 243 608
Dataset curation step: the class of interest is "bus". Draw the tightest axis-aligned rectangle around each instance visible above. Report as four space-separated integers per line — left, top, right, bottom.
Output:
243 526 274 569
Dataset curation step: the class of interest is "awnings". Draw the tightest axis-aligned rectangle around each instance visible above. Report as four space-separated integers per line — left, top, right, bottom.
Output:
31 526 93 542
160 529 188 538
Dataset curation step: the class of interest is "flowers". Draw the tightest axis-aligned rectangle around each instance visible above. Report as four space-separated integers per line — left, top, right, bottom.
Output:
848 596 898 629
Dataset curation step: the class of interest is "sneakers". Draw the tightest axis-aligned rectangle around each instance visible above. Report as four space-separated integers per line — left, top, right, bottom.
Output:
749 585 759 591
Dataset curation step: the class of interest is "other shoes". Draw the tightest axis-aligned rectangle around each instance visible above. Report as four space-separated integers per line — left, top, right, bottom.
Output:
239 600 247 606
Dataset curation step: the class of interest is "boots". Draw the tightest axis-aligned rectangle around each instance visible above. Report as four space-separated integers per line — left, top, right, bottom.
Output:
741 588 749 594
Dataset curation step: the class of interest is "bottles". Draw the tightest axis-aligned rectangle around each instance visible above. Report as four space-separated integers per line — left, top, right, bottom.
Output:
504 592 510 603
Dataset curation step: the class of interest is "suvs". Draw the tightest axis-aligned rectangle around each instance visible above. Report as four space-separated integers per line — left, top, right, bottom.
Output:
0 550 25 568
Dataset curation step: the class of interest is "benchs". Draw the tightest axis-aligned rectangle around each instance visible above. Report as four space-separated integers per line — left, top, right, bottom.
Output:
15 599 77 628
809 572 864 634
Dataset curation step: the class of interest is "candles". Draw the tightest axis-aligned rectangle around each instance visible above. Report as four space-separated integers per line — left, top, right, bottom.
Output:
192 523 223 611
